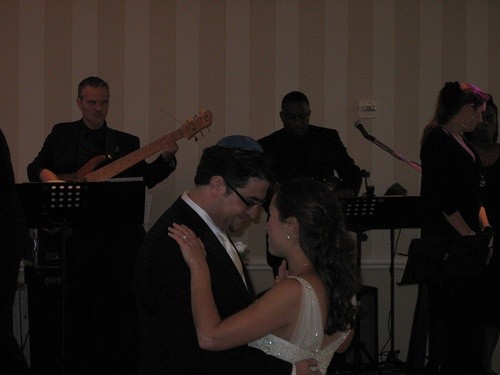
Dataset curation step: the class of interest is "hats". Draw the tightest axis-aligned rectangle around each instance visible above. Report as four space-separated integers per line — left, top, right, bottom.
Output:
217 135 263 152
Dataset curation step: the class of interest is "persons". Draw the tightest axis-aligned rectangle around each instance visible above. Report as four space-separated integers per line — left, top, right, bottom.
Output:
255 92 362 281
137 136 325 375
419 79 500 375
0 77 177 375
168 178 357 375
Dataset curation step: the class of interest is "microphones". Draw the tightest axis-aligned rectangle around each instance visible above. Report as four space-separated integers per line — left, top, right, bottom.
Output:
355 120 370 139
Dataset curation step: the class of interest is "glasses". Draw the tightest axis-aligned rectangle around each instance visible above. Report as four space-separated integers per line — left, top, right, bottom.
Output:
227 183 263 209
283 110 310 120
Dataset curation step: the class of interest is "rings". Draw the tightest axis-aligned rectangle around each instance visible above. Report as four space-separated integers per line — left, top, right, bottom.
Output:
182 235 189 240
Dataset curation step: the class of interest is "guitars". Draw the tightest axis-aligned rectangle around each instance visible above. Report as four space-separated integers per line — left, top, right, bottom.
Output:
34 106 213 181
